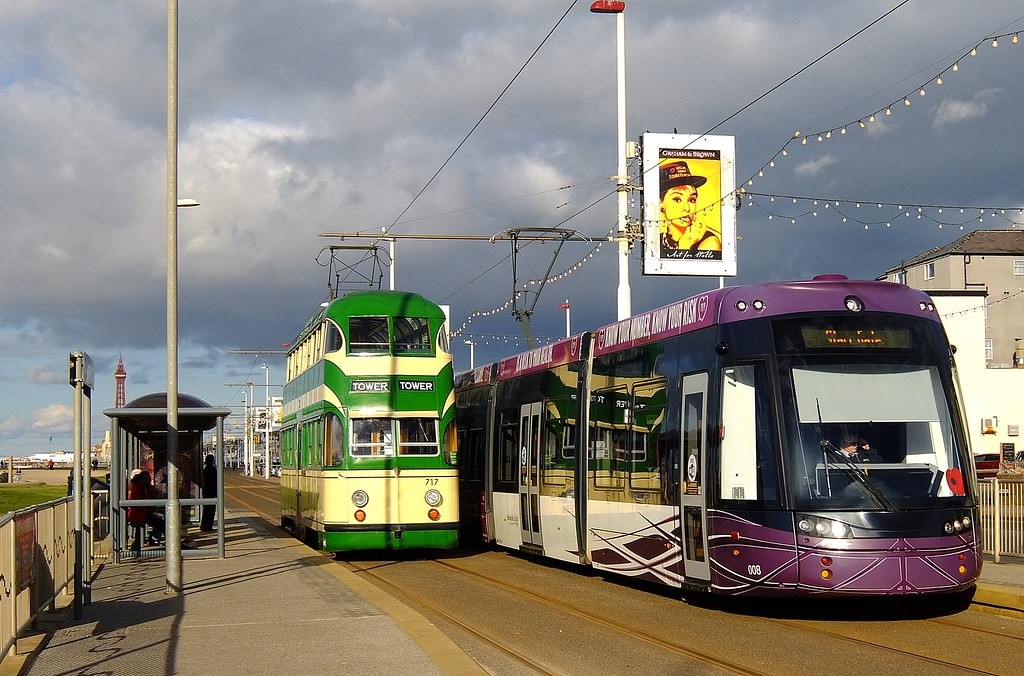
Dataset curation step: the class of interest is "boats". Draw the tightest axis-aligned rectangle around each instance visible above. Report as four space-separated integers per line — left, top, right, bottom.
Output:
28 450 95 463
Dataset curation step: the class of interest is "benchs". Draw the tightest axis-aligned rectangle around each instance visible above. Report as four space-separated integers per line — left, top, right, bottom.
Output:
126 521 149 551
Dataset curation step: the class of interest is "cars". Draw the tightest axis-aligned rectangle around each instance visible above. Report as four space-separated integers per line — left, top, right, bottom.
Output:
256 454 281 477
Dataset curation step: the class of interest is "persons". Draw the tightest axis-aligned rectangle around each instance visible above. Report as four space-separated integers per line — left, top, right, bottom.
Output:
660 161 722 252
92 453 217 546
829 430 884 464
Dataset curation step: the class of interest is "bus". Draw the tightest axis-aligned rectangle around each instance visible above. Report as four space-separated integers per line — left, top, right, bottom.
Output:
278 245 462 557
456 223 982 611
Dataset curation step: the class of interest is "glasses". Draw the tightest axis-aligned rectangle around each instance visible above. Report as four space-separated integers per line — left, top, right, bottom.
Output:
845 439 861 446
